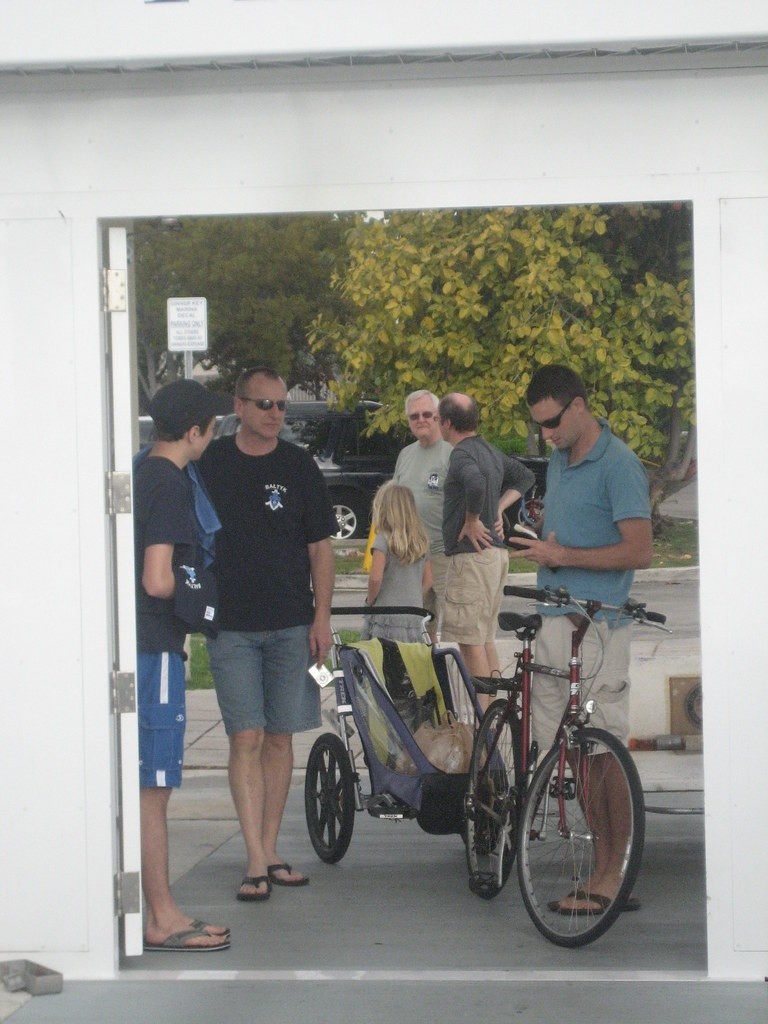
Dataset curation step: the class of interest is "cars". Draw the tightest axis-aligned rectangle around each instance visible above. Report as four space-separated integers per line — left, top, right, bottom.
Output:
212 400 550 545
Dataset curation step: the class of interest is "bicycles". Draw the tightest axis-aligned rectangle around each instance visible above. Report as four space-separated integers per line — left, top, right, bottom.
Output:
460 585 674 950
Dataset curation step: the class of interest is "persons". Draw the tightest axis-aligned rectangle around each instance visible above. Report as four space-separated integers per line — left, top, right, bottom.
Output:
199 367 340 900
133 380 230 951
362 485 434 645
437 393 535 746
508 364 653 915
393 390 453 650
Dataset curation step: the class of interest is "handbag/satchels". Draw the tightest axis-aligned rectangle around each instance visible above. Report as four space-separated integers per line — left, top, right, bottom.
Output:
396 710 478 774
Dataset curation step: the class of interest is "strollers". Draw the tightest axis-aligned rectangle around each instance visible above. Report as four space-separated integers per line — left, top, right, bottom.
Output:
303 605 511 865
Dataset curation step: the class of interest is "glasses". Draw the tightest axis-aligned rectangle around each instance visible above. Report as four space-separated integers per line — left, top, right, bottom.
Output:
532 401 572 429
409 411 434 420
235 397 289 411
432 415 445 421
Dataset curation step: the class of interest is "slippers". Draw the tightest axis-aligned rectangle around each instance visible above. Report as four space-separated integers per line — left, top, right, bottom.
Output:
143 928 230 952
549 889 584 910
267 864 309 886
188 919 231 938
561 894 640 915
237 876 271 901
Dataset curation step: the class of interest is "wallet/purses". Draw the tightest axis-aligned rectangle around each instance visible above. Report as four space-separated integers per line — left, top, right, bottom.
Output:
503 524 559 574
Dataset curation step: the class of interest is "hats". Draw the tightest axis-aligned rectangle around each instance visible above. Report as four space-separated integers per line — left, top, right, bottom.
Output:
148 379 234 433
